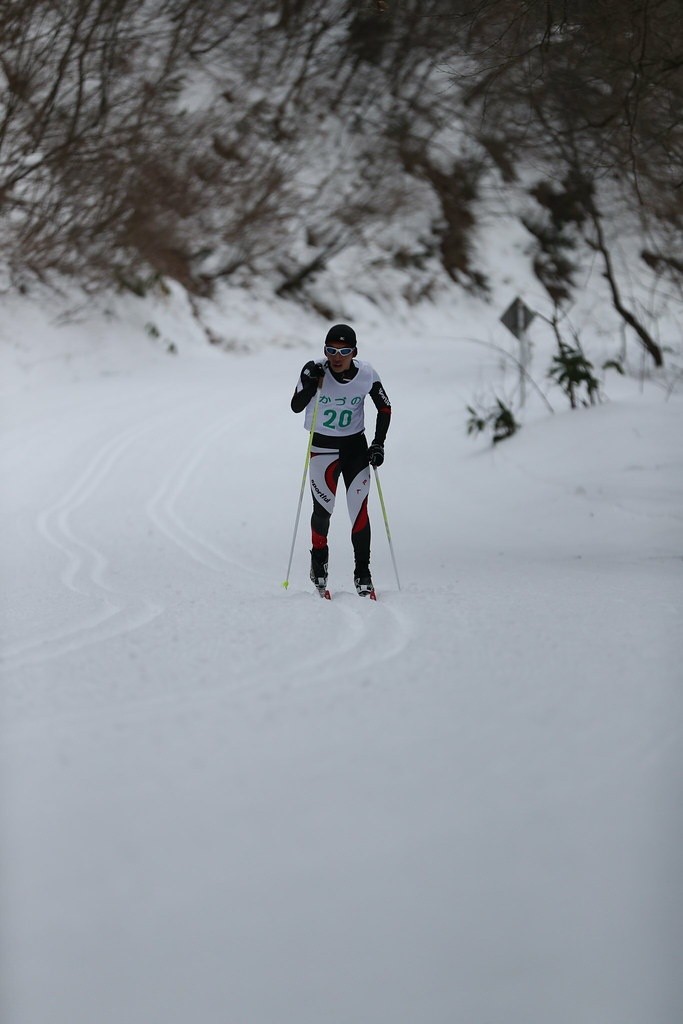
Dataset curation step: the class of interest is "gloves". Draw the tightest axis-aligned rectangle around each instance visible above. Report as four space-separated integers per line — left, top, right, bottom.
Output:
367 439 384 466
301 360 324 394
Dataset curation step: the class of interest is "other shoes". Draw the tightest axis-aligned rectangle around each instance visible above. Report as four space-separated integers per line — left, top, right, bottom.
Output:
354 578 373 596
311 578 327 597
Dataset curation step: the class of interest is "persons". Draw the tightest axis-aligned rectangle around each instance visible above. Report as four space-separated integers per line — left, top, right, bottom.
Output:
291 325 392 594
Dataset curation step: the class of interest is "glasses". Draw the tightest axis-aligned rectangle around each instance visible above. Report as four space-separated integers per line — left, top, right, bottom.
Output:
326 347 356 357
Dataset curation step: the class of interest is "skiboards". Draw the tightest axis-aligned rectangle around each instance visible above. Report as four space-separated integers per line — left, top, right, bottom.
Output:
311 577 377 601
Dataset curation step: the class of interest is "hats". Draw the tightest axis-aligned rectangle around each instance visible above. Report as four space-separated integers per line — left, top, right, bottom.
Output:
325 324 356 346
